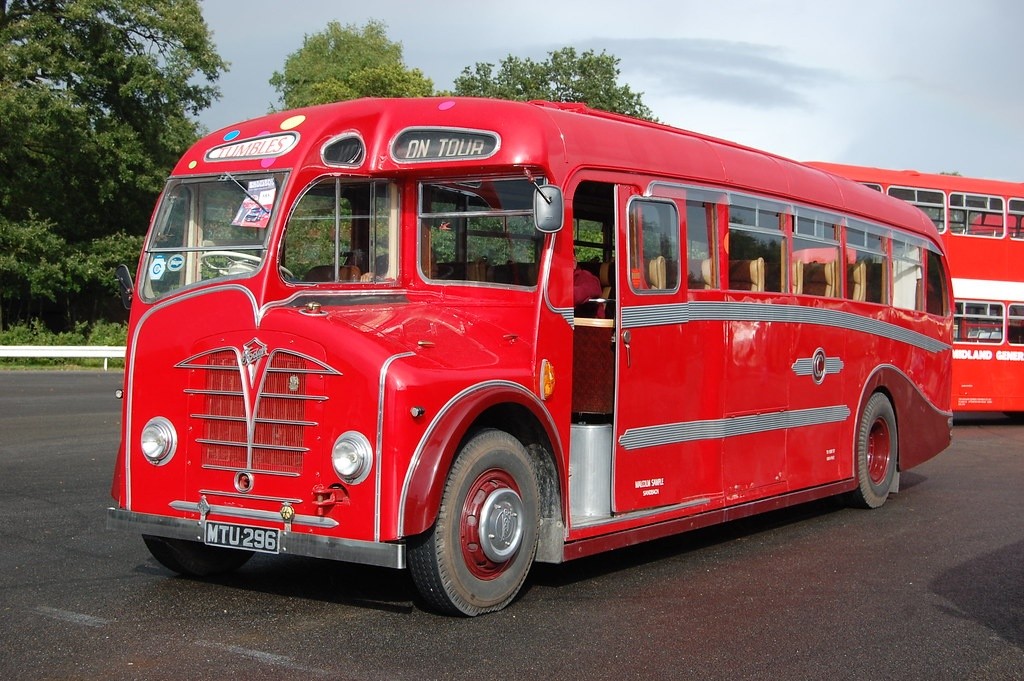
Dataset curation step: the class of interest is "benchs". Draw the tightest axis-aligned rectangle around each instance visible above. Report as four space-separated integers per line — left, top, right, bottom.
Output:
297 259 944 424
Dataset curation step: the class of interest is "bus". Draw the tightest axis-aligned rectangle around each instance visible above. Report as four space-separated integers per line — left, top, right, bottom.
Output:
800 162 1023 414
112 94 956 619
